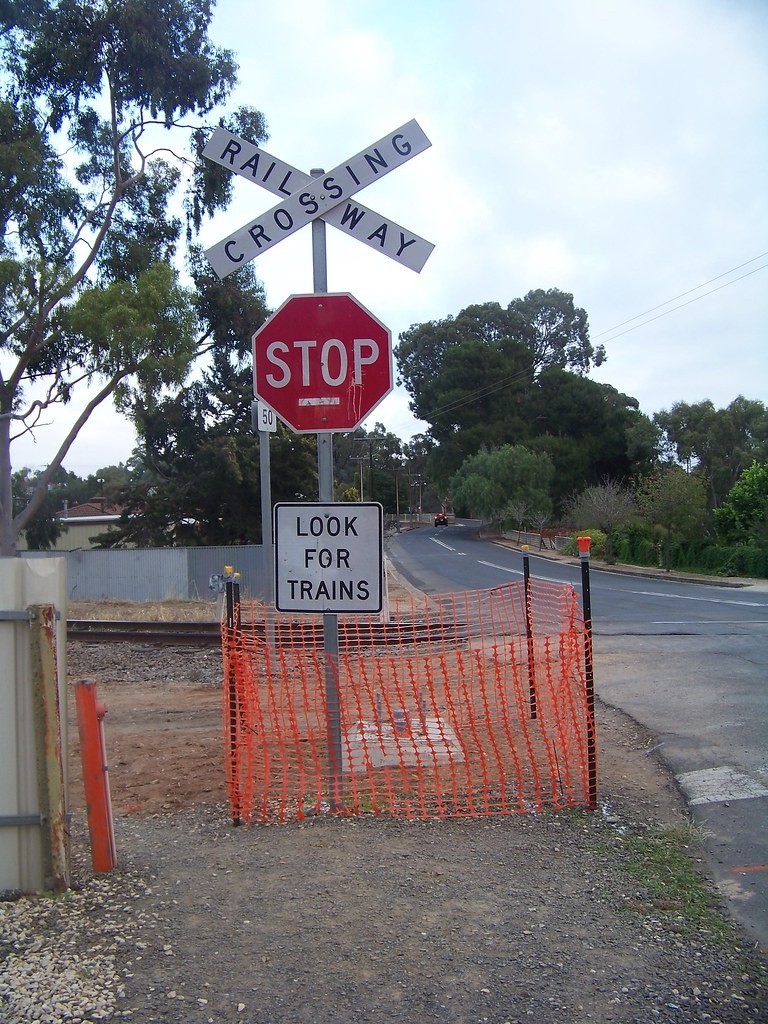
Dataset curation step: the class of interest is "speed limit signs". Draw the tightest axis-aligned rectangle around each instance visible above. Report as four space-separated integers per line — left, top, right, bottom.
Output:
252 400 277 433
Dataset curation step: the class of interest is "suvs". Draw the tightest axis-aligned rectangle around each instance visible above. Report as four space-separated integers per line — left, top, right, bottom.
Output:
434 513 448 527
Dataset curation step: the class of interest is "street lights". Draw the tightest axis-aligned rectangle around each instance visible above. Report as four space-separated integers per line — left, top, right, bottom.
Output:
97 477 105 515
412 481 427 513
417 472 422 523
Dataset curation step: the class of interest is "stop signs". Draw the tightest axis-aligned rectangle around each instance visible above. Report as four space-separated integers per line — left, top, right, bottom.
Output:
251 291 394 434
